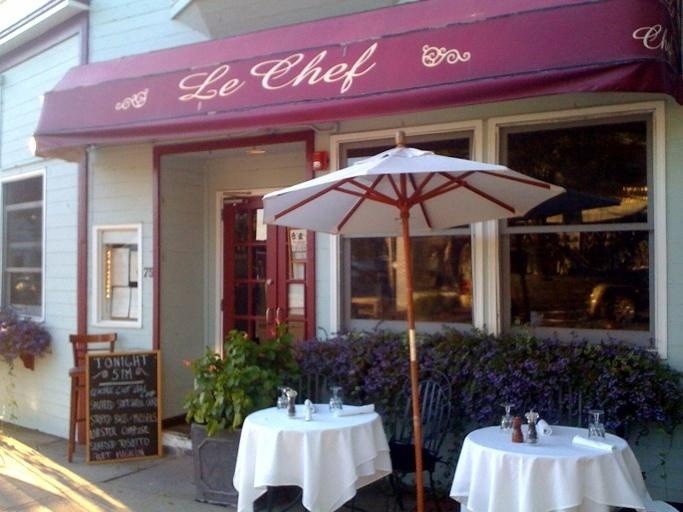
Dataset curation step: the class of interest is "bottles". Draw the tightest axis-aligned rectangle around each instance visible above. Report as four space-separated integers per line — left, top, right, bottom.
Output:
525 411 540 444
303 399 316 421
512 416 524 444
286 390 298 420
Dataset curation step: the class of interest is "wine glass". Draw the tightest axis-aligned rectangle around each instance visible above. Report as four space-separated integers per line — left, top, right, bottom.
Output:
499 403 516 433
587 409 606 442
328 386 344 413
276 386 290 410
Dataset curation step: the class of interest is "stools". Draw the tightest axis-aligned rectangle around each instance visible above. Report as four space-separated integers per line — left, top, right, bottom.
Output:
67 333 118 463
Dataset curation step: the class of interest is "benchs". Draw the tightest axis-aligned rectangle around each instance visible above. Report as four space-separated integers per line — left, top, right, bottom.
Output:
352 296 388 319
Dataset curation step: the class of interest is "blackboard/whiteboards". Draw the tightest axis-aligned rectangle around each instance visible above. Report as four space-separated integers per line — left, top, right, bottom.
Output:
84 349 163 465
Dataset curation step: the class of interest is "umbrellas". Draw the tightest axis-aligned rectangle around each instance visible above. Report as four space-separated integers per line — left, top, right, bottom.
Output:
262 131 567 512
511 154 647 325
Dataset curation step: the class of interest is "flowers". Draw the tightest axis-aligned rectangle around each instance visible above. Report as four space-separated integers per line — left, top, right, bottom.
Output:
0 304 52 421
